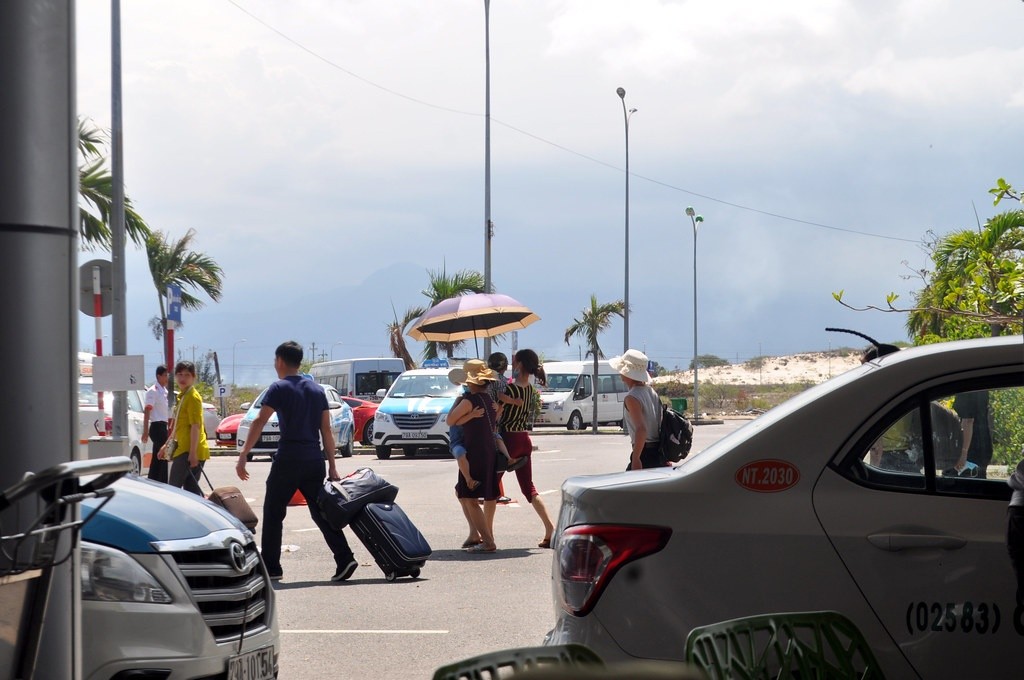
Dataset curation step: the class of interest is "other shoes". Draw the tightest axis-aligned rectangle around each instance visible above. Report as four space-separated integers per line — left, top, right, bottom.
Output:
467 479 480 492
507 456 528 473
497 496 512 502
267 565 283 580
331 559 358 581
477 497 485 504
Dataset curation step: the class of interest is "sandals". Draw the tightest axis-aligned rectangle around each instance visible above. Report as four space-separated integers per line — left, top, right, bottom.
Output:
539 535 550 547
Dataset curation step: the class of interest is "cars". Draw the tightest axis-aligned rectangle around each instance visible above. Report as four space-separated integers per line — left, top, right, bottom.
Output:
235 383 357 462
215 396 379 449
75 353 218 477
543 336 1024 680
375 355 468 461
0 472 281 680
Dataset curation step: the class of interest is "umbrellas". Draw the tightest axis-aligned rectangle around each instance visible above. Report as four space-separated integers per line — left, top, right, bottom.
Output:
406 294 542 357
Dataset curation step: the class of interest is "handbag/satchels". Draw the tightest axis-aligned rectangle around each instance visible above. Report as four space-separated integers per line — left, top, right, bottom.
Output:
494 453 507 472
161 438 179 461
317 468 398 531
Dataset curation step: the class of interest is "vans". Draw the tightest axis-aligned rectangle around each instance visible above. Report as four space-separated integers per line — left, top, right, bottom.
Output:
309 357 406 403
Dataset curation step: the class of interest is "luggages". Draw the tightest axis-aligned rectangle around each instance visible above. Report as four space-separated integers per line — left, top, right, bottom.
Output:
327 476 432 582
185 461 258 534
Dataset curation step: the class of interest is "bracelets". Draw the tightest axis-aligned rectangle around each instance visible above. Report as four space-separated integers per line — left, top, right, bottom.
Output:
962 448 968 450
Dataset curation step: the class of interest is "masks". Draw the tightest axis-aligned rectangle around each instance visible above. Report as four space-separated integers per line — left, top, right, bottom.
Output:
513 364 520 379
958 460 978 478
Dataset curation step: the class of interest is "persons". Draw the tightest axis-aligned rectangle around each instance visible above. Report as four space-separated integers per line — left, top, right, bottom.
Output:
142 365 169 483
156 361 209 496
236 341 358 582
445 349 555 554
609 349 672 470
861 344 995 479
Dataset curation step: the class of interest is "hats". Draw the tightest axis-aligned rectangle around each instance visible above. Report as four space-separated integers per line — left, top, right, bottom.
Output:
462 384 471 393
488 352 508 372
608 349 652 383
448 359 500 386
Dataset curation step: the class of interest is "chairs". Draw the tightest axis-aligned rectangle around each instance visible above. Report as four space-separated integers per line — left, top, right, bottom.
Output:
434 612 888 680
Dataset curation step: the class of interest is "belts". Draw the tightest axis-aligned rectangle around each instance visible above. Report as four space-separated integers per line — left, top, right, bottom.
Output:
883 449 906 454
157 421 167 425
632 442 659 449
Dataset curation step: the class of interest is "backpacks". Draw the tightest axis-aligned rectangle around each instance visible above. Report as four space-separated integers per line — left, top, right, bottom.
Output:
657 397 693 463
911 401 962 470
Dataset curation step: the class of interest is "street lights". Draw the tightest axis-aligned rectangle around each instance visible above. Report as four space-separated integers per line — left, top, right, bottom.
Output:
232 338 246 391
330 342 343 360
684 205 704 425
615 85 638 360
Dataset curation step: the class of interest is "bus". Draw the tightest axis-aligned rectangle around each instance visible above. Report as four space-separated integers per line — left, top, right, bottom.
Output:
536 360 630 431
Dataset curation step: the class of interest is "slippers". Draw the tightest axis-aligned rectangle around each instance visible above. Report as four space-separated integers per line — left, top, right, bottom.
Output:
463 539 480 548
467 544 496 554
